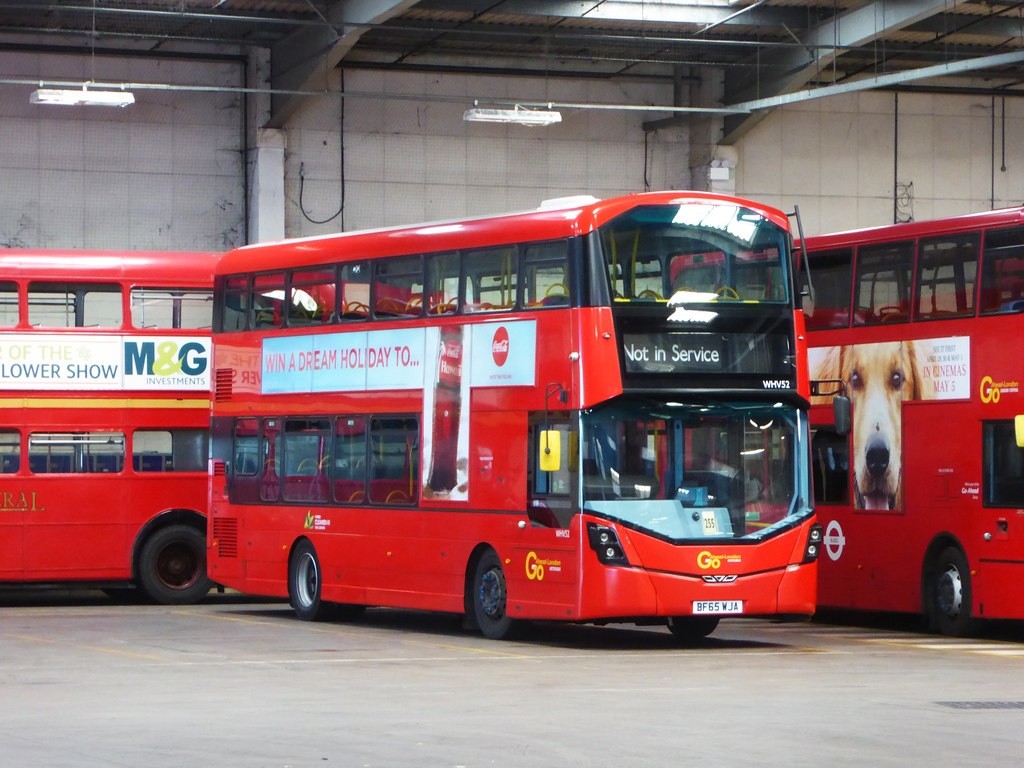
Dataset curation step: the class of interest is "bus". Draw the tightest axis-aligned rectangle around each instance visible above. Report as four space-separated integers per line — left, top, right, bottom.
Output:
205 188 853 645
1 243 423 608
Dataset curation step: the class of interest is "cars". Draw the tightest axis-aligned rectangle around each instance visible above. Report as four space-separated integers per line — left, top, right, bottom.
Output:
645 200 1023 637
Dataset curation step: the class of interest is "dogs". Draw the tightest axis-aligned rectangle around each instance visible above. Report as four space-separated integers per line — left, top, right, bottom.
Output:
810 341 941 511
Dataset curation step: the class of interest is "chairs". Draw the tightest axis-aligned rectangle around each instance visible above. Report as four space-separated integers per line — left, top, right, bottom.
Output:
0 451 165 473
256 284 740 332
257 457 417 504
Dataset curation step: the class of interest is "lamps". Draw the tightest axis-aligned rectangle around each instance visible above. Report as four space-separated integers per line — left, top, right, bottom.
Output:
29 89 135 107
463 109 563 126
